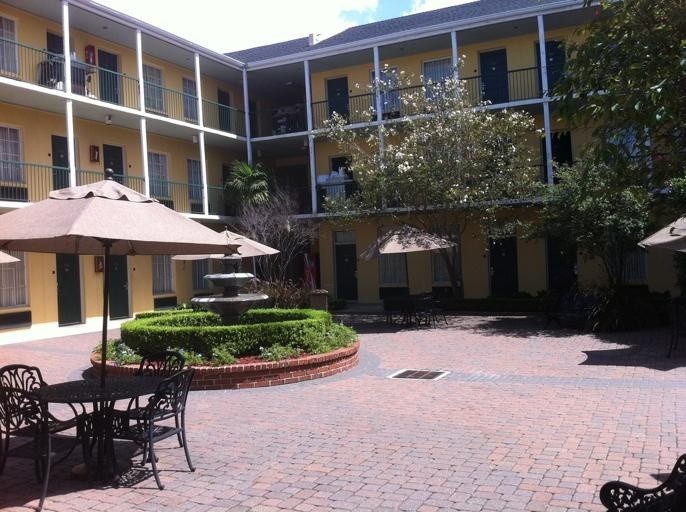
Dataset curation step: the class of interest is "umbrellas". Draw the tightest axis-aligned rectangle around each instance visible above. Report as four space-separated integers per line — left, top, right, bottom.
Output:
171 223 282 261
637 213 686 255
1 168 242 384
358 221 460 292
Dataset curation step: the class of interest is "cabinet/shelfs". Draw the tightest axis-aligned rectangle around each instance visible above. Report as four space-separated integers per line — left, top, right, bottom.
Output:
384 288 448 329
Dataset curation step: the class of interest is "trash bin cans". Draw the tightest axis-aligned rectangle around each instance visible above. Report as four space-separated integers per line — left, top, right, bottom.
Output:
309 289 328 310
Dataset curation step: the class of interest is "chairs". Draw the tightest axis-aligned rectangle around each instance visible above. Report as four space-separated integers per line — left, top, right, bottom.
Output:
1 349 195 511
599 453 686 512
666 296 686 358
